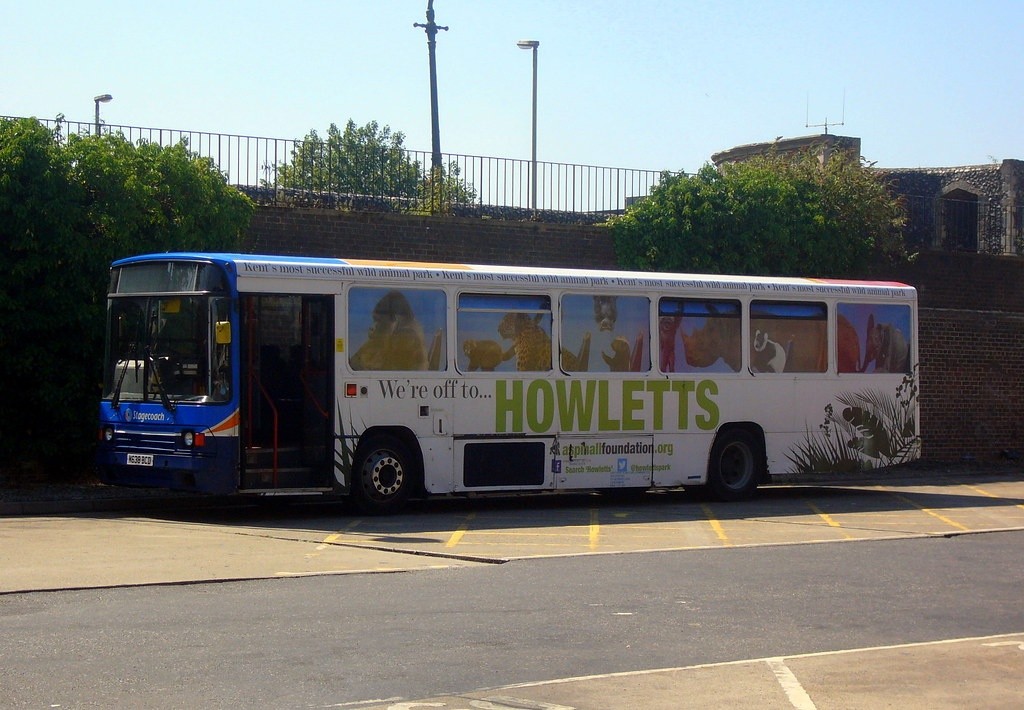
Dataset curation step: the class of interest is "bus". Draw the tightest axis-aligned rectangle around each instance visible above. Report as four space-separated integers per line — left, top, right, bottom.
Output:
93 251 922 516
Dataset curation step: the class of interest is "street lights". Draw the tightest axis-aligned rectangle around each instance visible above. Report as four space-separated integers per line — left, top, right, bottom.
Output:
94 95 112 133
518 38 538 210
